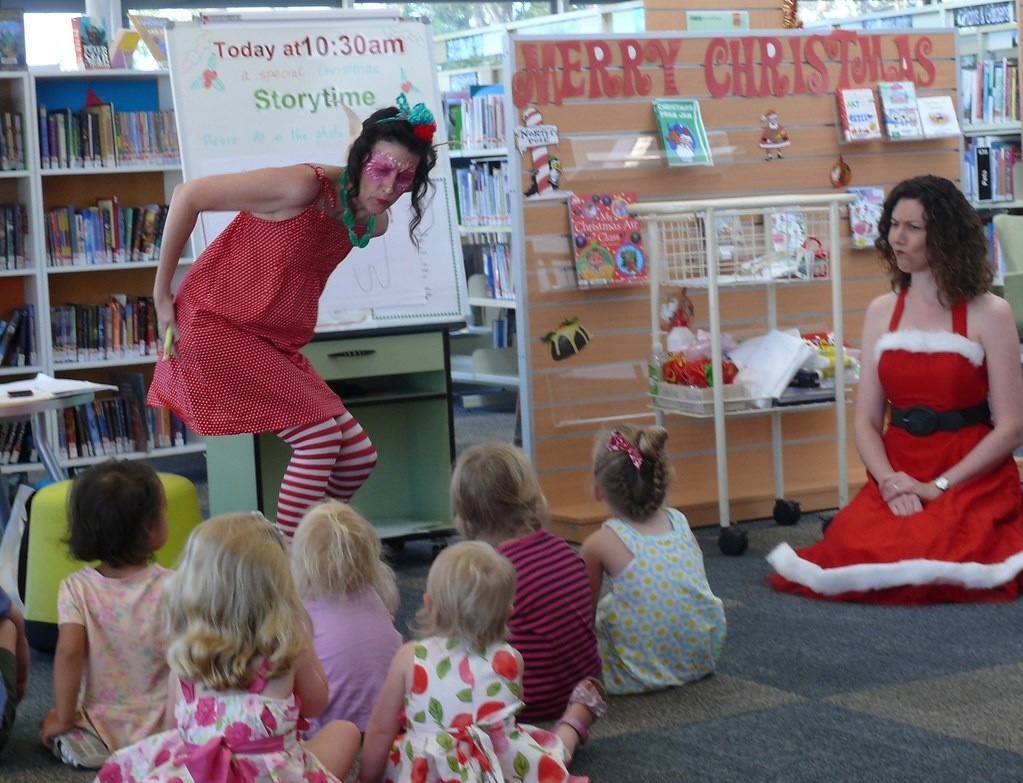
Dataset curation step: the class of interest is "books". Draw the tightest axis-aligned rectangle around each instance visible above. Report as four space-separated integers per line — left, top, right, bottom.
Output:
651 99 715 167
439 84 516 349
568 192 648 288
0 10 186 519
836 57 1022 279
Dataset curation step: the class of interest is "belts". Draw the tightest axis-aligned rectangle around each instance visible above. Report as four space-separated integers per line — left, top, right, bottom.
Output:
886 404 990 437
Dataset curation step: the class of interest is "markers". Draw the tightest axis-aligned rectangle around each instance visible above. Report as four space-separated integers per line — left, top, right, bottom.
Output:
162 327 173 361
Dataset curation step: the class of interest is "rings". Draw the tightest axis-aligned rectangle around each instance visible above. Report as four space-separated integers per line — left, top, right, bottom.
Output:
892 482 900 490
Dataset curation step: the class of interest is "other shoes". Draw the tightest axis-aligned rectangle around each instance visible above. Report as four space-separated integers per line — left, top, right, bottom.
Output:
50 726 110 768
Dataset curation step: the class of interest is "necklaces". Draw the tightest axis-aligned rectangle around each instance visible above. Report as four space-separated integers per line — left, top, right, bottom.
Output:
340 166 375 249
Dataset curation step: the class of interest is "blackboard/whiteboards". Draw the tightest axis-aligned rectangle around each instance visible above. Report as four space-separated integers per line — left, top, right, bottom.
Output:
167 16 472 333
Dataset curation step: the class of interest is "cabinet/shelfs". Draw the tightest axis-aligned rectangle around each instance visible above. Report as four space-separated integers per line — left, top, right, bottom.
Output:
448 24 964 546
806 0 1023 287
624 192 862 558
0 70 53 474
28 69 208 478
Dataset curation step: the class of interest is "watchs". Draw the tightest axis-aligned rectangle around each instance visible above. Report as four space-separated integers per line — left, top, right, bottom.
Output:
932 476 950 492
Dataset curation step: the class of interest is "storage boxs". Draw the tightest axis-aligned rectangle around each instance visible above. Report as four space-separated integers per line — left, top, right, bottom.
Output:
657 382 752 414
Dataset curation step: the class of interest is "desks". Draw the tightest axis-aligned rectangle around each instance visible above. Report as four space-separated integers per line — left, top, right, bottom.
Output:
0 381 95 482
204 322 468 557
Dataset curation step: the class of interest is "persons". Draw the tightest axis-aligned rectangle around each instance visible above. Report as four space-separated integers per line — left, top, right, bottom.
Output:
584 249 614 280
618 251 639 274
0 423 725 783
147 93 437 550
765 173 1023 603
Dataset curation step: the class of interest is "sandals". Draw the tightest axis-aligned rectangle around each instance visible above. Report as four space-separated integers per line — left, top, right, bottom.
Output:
548 677 610 747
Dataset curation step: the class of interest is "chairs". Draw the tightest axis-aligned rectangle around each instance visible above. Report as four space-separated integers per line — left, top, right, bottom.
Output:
993 214 1023 340
